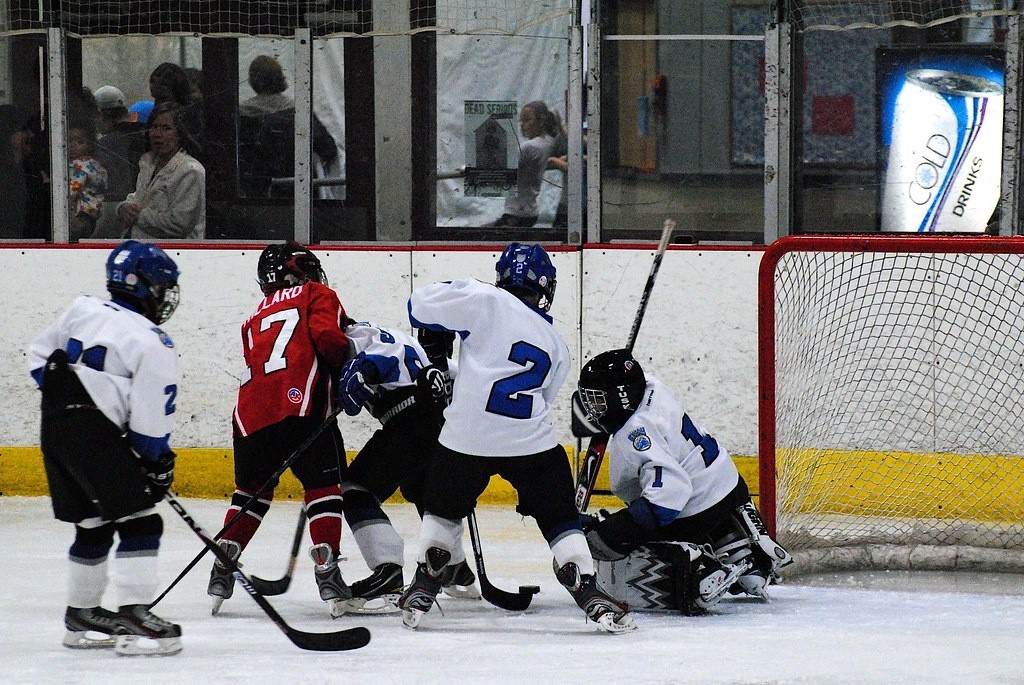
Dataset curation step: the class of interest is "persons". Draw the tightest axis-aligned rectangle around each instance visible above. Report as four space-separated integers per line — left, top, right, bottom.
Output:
329 313 484 616
395 242 637 632
510 97 591 207
240 55 338 204
574 345 797 614
208 241 352 620
25 238 190 657
0 61 231 239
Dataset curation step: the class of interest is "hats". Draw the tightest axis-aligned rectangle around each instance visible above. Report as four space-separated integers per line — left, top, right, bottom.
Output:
93 85 127 108
128 101 155 123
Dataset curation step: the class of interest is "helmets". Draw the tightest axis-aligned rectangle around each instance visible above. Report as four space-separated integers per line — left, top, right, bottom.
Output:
106 240 179 300
496 242 557 304
258 242 321 290
578 349 646 434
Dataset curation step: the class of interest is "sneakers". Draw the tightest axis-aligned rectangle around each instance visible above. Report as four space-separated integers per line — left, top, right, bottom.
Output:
309 543 352 617
345 562 405 614
553 557 638 634
62 606 118 650
399 547 455 631
207 539 241 614
684 542 748 609
727 535 794 595
115 604 183 659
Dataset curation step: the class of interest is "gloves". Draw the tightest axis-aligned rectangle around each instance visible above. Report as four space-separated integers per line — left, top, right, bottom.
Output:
141 452 178 503
417 328 456 371
581 508 644 561
337 357 381 416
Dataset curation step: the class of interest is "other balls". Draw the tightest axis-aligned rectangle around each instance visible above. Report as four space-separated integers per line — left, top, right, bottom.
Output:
519 585 541 595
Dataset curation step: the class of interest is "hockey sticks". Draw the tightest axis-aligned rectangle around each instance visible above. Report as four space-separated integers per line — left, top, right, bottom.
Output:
122 431 371 652
148 405 344 610
552 219 677 614
441 355 533 612
250 499 305 597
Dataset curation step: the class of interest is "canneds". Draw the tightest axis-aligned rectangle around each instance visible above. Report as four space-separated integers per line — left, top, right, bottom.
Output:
880 70 1005 233
